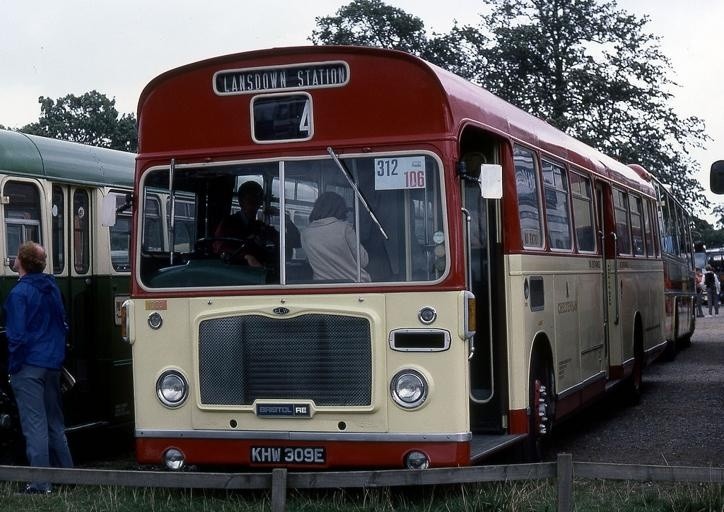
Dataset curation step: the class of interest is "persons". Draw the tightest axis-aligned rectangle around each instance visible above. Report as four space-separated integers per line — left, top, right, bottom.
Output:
353 183 419 282
695 264 723 318
5 239 77 495
298 190 373 282
212 180 302 283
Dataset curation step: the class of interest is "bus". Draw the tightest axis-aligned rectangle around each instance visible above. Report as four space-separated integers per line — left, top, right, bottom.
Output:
0 129 321 489
626 164 698 350
117 43 668 476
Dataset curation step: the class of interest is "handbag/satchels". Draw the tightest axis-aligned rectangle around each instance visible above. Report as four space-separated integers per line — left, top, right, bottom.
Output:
57 362 77 396
